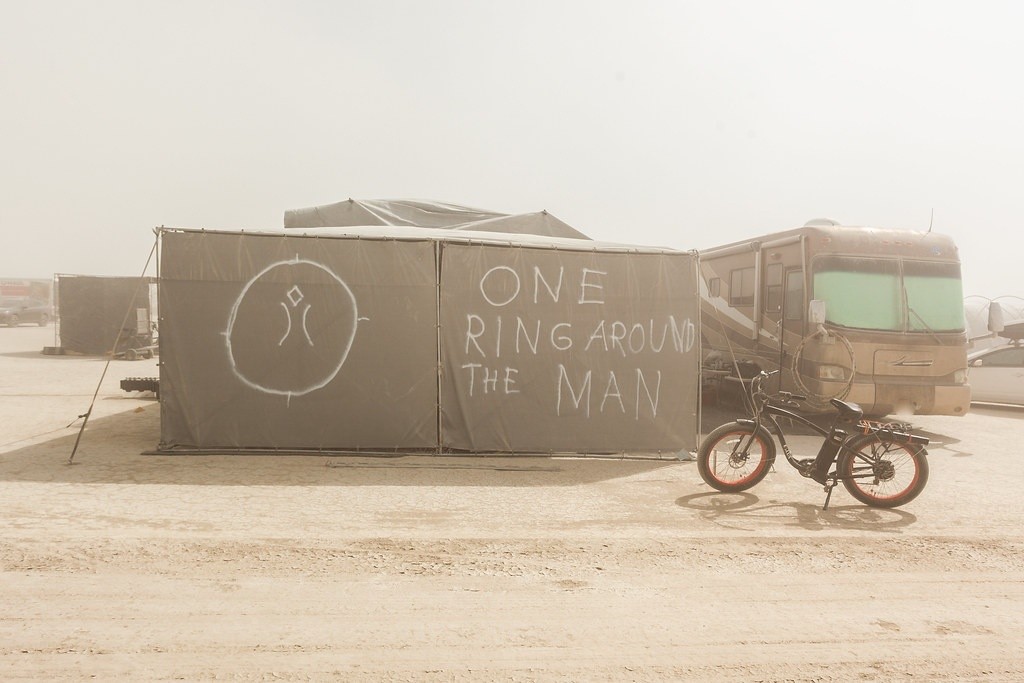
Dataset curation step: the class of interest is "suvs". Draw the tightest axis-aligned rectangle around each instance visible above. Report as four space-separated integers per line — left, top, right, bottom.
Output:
967 319 1024 409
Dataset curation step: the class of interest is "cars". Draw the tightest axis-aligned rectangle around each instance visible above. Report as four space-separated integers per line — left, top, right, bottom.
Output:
0 295 51 327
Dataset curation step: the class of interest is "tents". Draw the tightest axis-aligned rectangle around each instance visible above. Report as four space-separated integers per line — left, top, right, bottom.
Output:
127 193 712 467
47 266 165 364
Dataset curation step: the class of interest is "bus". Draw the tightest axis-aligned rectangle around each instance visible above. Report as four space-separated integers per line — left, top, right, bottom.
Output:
699 218 1004 424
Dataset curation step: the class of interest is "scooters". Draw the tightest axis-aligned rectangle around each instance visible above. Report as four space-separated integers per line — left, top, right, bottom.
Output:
698 359 930 512
